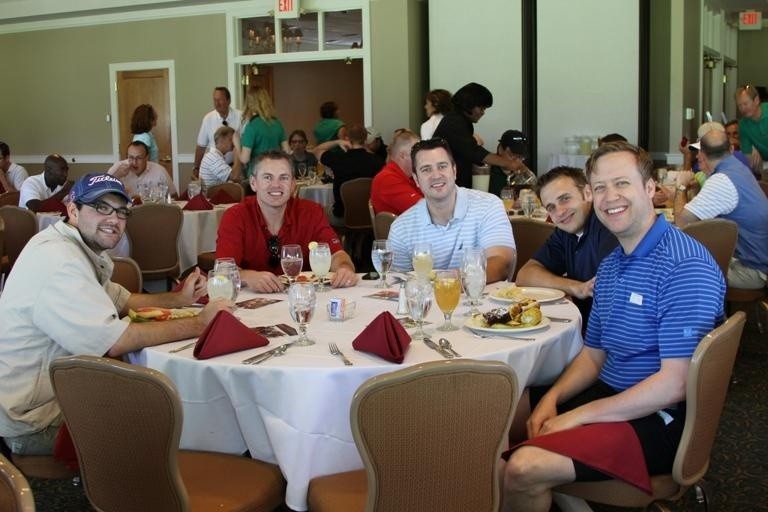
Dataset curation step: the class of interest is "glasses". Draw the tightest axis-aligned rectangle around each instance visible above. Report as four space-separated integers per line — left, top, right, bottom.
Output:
250 172 293 183
411 136 447 150
222 121 227 126
267 235 281 269
75 199 132 219
127 154 148 162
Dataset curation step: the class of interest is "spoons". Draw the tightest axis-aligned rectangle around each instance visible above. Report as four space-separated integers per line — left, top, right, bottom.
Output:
439 338 462 357
252 344 288 366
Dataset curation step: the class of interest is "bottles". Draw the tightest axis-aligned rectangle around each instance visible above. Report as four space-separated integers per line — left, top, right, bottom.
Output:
329 297 345 322
397 280 406 315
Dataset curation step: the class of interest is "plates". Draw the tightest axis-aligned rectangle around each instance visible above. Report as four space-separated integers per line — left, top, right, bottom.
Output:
464 315 552 333
489 286 565 304
121 302 205 323
278 275 331 285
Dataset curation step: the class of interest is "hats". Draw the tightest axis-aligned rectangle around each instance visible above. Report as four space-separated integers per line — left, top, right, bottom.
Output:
68 172 133 204
498 130 527 155
686 141 700 150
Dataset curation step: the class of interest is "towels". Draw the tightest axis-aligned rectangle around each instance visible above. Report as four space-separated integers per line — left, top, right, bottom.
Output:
193 309 269 359
500 422 652 496
351 311 412 364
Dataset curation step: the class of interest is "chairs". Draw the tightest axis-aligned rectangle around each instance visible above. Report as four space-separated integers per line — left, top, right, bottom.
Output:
340 176 396 241
682 218 740 385
0 436 69 479
0 454 36 512
728 288 767 333
50 355 285 511
554 310 746 512
306 358 518 511
0 183 243 293
511 219 554 270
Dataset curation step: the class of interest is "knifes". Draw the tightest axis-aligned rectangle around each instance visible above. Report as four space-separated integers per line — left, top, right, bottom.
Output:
423 338 454 358
242 342 294 365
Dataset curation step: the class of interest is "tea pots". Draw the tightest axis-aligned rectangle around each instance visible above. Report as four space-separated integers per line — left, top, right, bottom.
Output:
505 169 538 201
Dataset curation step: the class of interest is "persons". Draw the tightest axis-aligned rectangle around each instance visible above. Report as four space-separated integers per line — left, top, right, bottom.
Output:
17 153 75 213
516 167 617 337
104 83 625 225
215 151 358 293
0 140 29 193
375 138 516 291
653 84 767 290
1 174 236 458
500 140 727 512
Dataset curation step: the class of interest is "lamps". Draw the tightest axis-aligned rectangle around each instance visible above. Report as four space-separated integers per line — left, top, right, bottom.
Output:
245 18 302 52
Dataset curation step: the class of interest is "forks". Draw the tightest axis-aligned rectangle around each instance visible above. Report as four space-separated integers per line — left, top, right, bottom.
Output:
329 344 353 366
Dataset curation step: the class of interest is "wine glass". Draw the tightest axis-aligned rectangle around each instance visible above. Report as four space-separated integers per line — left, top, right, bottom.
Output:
187 183 200 200
463 254 485 318
519 188 532 207
297 163 307 183
213 257 235 272
136 176 170 203
371 238 394 289
230 267 241 303
308 242 332 292
412 242 433 295
317 162 326 180
280 243 304 286
307 165 317 186
522 192 537 218
191 181 202 190
434 270 462 332
501 189 515 220
405 276 433 340
288 281 317 346
463 245 487 306
207 269 240 320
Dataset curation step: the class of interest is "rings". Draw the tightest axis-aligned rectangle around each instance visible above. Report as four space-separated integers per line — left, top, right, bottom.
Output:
346 280 352 283
